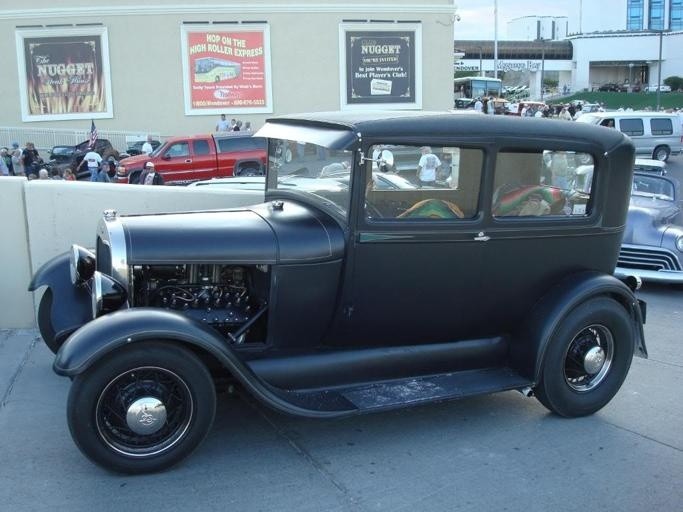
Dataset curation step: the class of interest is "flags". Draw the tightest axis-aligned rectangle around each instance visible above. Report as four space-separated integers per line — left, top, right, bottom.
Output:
87 121 98 149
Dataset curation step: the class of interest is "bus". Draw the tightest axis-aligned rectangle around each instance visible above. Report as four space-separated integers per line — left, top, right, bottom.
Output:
454 77 502 109
194 57 241 82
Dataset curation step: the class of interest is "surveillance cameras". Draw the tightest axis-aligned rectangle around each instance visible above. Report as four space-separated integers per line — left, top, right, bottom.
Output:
456 16 461 22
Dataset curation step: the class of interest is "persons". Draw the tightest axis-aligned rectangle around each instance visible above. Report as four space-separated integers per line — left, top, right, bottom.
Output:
539 147 596 196
214 113 252 132
474 93 682 129
416 147 441 187
377 144 393 172
0 133 165 186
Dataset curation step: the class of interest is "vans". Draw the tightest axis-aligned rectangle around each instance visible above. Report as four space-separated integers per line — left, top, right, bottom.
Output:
574 112 683 161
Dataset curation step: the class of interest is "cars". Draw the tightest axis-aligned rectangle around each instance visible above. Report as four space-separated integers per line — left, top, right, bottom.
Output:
482 98 604 119
570 166 683 289
26 105 649 476
35 137 121 180
598 82 672 93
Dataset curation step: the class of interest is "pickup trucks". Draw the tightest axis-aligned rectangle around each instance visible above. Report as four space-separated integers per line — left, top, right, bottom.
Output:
114 130 268 185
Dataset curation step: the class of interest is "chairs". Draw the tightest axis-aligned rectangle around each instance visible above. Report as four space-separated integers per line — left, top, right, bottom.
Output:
393 199 465 220
499 182 570 220
